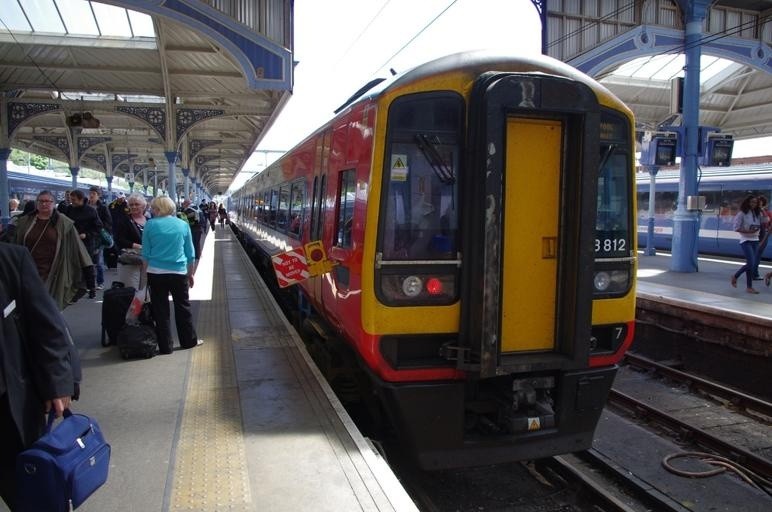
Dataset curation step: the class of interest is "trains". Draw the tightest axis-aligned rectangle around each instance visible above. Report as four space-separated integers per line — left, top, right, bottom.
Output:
222 48 638 470
596 159 625 231
287 193 354 244
8 169 157 220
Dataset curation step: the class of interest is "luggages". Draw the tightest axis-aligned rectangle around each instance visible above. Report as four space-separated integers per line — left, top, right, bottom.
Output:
99 280 138 340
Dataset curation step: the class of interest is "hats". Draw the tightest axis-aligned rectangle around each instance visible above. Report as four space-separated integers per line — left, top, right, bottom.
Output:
182 199 193 209
115 190 125 198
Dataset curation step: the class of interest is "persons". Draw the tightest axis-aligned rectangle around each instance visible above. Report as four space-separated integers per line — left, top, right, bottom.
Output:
751 195 772 281
731 194 763 294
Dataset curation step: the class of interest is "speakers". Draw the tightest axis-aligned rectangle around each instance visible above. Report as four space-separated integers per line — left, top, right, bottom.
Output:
687 196 697 210
697 196 705 209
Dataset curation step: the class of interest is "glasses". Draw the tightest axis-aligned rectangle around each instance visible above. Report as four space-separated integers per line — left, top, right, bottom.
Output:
36 200 53 205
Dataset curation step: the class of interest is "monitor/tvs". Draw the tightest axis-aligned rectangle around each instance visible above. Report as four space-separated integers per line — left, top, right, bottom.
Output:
711 139 734 167
655 138 677 166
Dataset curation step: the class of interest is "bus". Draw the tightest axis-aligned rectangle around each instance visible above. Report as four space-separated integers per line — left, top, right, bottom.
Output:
634 164 772 263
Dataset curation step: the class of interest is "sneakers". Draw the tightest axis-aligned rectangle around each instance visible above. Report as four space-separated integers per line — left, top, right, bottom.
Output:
192 337 204 347
95 281 104 290
87 288 98 301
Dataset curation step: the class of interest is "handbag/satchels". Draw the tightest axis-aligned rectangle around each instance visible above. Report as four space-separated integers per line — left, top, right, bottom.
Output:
116 321 158 359
125 282 159 328
16 402 112 512
